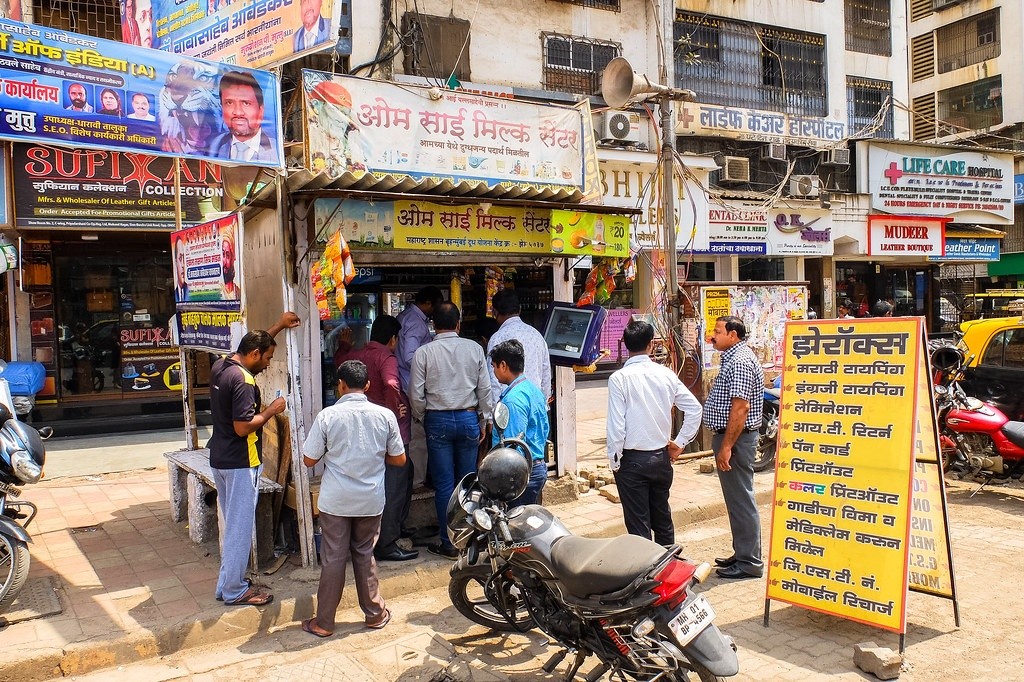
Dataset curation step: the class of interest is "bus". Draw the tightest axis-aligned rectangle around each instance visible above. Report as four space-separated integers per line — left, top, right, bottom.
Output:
961 289 1024 322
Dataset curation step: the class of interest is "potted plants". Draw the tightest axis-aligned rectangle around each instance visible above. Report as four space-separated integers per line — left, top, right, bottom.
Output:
943 60 960 73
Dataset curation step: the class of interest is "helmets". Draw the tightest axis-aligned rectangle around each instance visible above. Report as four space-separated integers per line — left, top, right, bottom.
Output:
931 343 965 372
477 437 533 501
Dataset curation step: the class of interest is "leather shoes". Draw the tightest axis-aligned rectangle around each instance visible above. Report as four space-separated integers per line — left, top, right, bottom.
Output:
715 564 757 579
374 543 419 561
399 527 417 538
715 554 738 567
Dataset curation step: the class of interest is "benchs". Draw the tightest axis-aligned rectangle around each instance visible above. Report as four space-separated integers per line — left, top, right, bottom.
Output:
158 447 284 573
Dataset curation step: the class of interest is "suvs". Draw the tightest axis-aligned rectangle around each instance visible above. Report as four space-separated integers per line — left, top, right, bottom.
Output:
895 290 913 304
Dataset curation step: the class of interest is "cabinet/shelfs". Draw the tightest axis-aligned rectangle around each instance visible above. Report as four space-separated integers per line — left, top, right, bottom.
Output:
23 240 62 401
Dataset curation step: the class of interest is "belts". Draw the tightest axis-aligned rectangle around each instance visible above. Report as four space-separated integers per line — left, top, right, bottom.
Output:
711 429 757 436
533 458 545 465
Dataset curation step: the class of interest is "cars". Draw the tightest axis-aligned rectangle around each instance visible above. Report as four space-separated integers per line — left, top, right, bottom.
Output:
59 319 160 367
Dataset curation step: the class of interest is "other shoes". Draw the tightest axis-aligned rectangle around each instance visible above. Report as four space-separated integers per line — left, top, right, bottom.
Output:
426 542 459 560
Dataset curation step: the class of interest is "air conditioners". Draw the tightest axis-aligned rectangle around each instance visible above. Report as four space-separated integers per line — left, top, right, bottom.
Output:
760 142 787 162
719 154 750 183
601 108 641 143
821 147 851 167
789 173 821 198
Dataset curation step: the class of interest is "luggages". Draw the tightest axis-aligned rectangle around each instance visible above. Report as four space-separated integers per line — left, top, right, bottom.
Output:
28 291 54 310
83 269 112 290
30 316 54 336
22 261 53 285
85 289 114 312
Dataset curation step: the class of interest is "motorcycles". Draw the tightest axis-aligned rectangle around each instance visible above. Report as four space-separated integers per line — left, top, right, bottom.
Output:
446 402 739 682
63 342 120 393
931 330 1024 499
0 403 53 615
752 374 781 471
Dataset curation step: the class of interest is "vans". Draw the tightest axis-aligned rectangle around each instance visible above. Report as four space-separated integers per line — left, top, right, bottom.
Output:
940 297 964 327
948 316 1024 421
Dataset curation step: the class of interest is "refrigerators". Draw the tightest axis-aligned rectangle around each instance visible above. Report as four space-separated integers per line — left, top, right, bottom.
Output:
320 267 383 411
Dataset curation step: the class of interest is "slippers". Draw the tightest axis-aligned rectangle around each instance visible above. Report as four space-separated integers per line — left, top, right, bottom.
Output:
224 589 274 606
302 617 333 637
216 577 253 601
367 607 394 629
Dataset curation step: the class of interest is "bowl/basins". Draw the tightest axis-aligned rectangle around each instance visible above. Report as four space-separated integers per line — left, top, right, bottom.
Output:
551 238 564 251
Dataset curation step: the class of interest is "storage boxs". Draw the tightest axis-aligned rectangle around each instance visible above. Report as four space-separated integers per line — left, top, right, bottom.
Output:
286 475 319 519
292 519 321 554
196 351 211 385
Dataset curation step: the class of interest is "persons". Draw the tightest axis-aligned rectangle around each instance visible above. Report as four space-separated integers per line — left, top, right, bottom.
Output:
222 221 240 300
311 83 352 160
68 83 152 120
874 301 894 317
606 320 703 545
122 0 152 47
174 235 188 302
325 298 371 379
839 303 854 318
410 302 492 558
333 314 419 559
208 0 228 15
185 222 220 245
303 360 406 637
704 317 764 577
211 72 273 160
209 312 302 605
490 339 549 610
487 289 552 434
291 0 331 53
848 275 868 316
393 285 445 489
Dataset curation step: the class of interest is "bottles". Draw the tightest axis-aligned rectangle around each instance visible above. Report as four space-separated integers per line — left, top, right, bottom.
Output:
384 211 391 244
519 289 551 310
325 370 342 406
341 305 362 319
325 333 338 359
595 216 605 253
